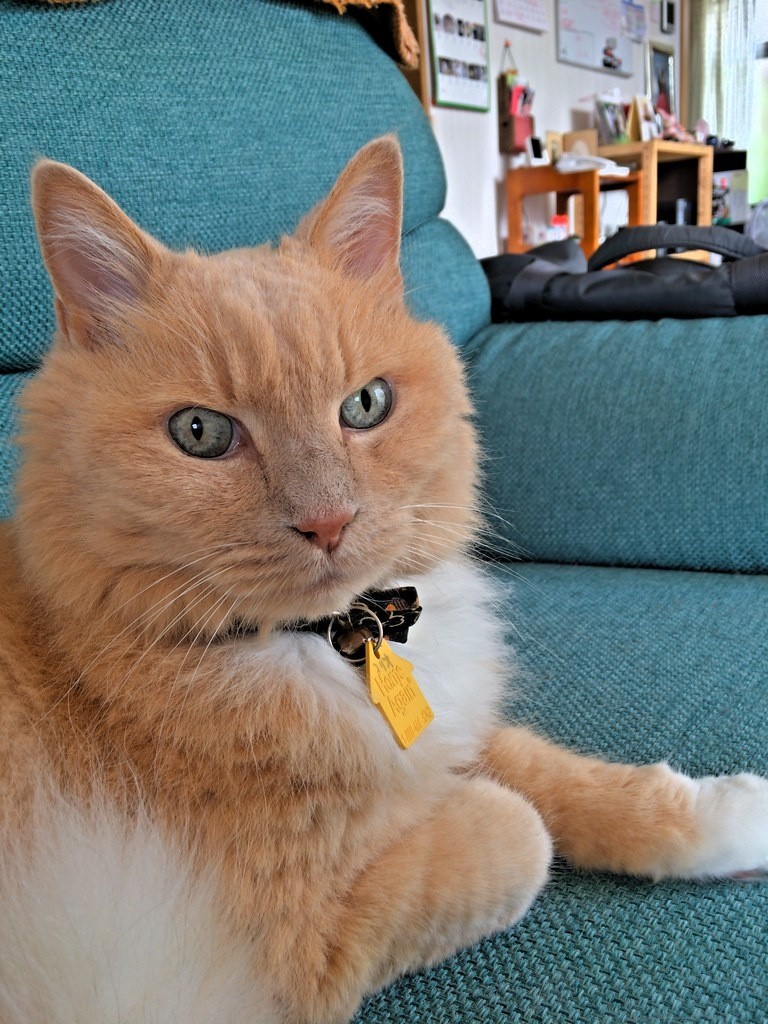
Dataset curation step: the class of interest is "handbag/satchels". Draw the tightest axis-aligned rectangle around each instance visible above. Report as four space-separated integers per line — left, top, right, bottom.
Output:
481 226 768 321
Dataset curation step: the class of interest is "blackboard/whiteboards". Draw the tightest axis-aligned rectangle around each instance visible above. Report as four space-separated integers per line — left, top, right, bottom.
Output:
553 0 633 78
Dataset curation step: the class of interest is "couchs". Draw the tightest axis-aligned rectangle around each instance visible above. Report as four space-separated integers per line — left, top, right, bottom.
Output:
0 1 767 1023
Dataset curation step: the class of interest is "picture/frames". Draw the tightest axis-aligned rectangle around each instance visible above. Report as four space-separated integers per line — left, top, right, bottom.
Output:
644 40 678 118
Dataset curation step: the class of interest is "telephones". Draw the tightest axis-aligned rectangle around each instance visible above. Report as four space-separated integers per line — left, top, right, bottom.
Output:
556 154 630 177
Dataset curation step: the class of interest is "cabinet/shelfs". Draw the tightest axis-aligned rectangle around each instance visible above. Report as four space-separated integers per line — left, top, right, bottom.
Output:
505 139 714 271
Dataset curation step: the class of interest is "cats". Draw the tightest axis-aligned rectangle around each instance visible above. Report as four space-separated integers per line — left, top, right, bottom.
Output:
0 133 766 1024
